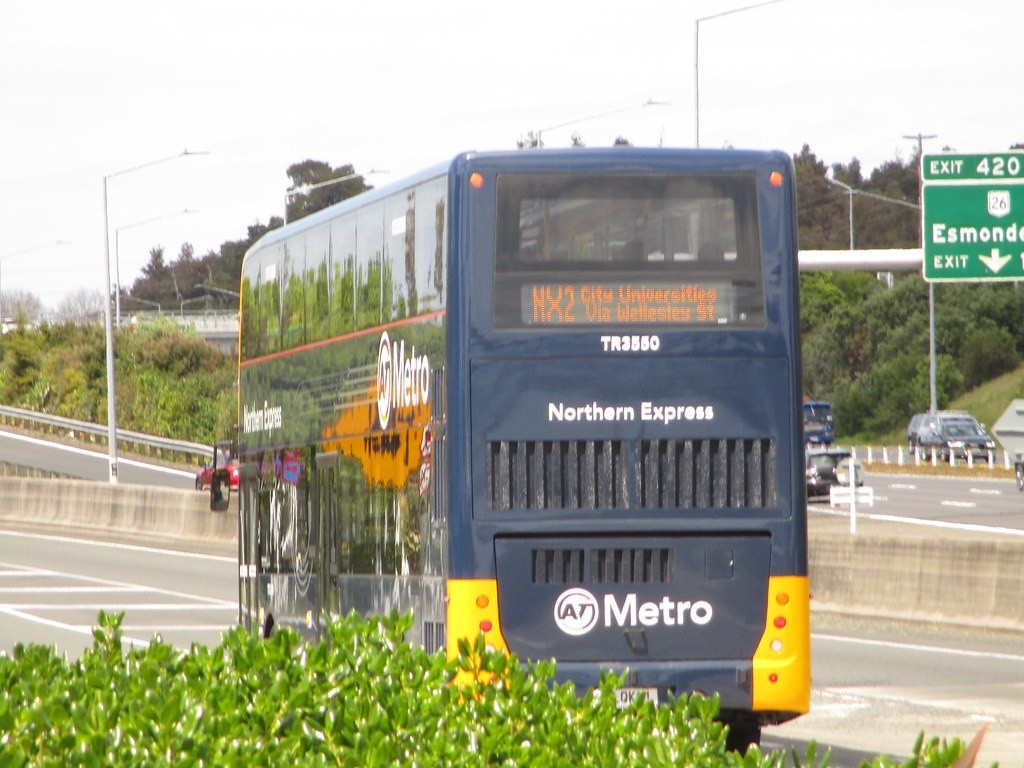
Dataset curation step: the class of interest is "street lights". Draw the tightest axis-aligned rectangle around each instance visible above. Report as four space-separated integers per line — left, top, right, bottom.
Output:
903 133 938 249
99 145 209 475
843 189 861 250
114 210 201 329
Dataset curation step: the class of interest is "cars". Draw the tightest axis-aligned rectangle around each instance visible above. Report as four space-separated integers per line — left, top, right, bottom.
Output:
906 410 998 464
195 453 238 492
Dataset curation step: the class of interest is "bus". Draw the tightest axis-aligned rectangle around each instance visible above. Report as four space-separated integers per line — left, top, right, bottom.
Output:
206 145 811 760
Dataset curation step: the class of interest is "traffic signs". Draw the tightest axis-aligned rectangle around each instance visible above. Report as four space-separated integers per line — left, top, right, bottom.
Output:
922 183 1024 282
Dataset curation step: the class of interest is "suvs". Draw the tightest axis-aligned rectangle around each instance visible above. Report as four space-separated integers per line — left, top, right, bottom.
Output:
806 449 865 498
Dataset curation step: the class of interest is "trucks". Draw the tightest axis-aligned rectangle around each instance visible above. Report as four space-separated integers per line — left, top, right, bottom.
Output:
804 401 835 447
